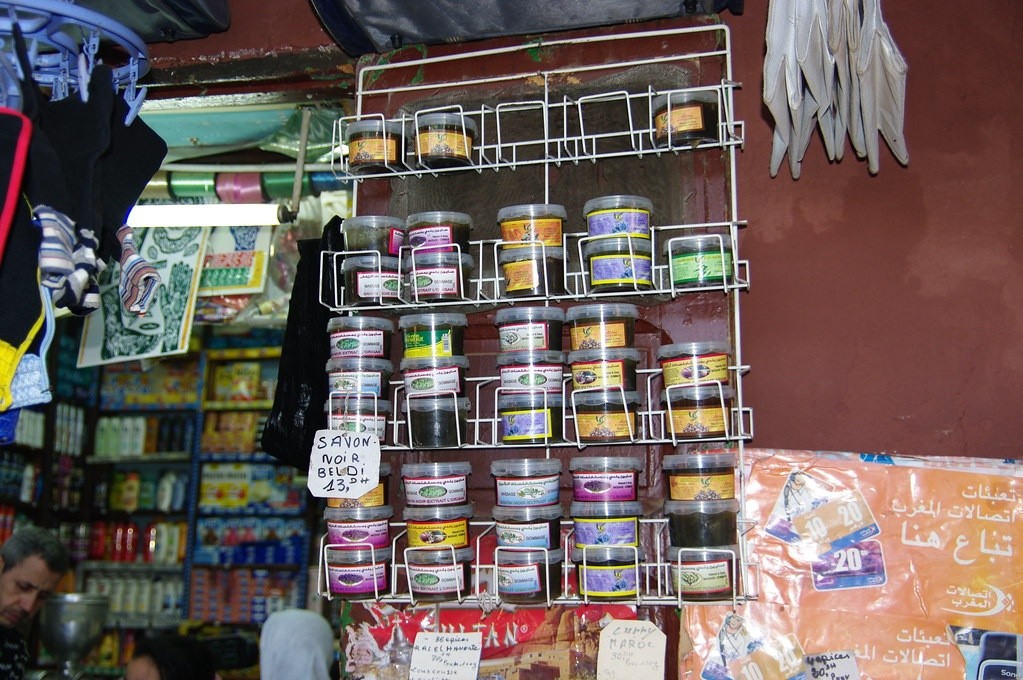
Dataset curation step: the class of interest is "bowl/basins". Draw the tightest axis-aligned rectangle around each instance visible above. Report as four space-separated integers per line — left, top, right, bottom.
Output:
322 93 739 604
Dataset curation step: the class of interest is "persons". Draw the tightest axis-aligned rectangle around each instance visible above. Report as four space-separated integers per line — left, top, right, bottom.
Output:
0 527 72 680
123 632 220 680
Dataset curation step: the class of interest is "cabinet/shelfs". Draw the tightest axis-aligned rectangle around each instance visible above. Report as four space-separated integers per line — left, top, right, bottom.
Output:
0 316 321 646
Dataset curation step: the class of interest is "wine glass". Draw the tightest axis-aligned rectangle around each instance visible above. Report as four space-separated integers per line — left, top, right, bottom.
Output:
39 592 111 680
